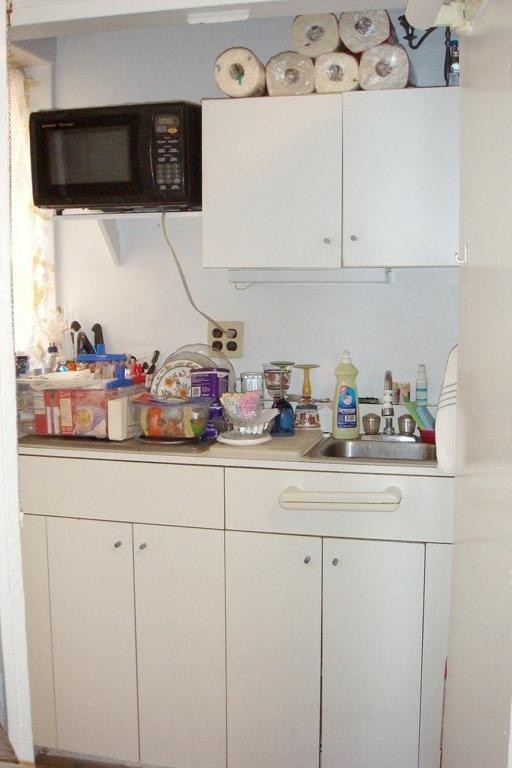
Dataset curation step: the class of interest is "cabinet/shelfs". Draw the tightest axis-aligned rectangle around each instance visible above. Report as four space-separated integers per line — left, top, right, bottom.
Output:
17 513 224 768
202 85 459 269
224 466 454 544
225 523 455 766
19 457 224 530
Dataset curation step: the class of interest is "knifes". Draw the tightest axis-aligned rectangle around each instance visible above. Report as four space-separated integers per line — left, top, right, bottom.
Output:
71 320 105 355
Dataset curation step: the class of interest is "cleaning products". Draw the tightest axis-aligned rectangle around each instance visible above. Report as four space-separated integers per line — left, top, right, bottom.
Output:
332 350 361 438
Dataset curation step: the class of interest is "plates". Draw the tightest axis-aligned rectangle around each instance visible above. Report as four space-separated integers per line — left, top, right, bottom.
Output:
150 343 236 409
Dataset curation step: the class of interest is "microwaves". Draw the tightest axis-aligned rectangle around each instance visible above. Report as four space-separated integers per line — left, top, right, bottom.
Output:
27 101 198 218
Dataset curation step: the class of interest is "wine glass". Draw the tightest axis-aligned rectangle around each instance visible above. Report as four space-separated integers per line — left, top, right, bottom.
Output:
264 360 324 438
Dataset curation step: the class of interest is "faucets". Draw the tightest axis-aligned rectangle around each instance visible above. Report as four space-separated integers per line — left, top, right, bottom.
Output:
381 368 396 435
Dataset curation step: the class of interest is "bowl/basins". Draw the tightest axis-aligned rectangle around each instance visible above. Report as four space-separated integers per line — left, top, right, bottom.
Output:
417 426 435 444
131 401 209 439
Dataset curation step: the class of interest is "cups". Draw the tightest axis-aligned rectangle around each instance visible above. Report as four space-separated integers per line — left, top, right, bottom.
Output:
217 393 273 440
233 371 264 394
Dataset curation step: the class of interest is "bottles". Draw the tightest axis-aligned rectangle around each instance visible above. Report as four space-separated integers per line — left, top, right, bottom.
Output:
53 356 70 372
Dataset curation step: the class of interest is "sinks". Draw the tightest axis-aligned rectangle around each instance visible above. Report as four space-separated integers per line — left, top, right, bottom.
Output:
303 435 437 468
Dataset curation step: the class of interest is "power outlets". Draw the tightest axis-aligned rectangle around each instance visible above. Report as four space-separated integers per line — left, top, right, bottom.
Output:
207 320 243 359
